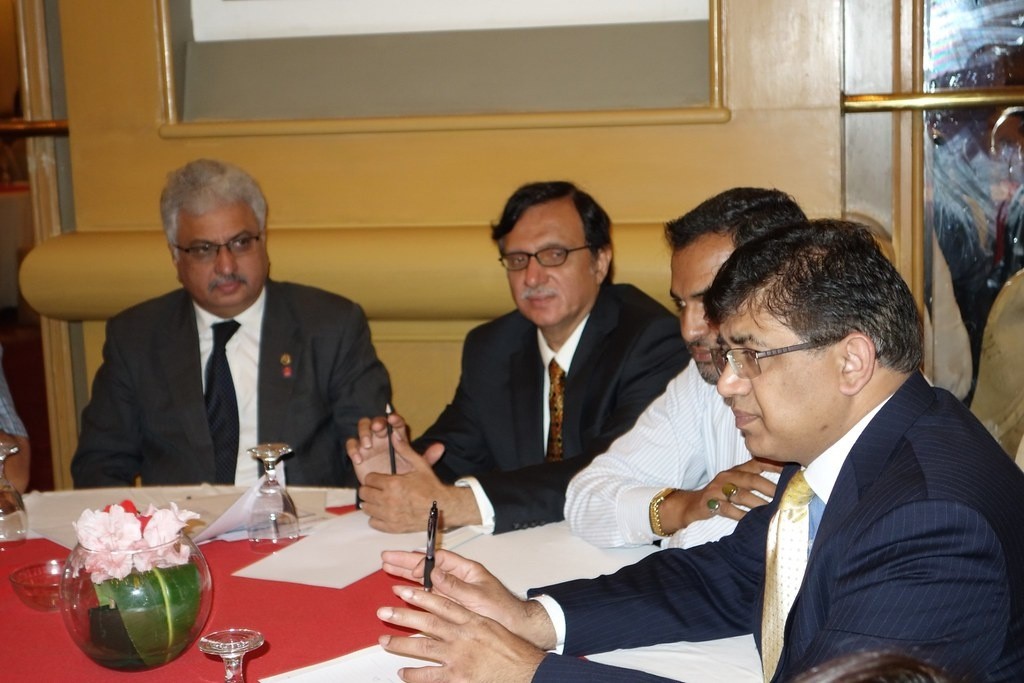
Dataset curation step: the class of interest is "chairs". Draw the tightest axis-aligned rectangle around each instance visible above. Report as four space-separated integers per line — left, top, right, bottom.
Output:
969 266 1024 460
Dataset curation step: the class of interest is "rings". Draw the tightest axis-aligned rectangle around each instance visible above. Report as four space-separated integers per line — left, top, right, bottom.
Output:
721 482 737 501
707 498 719 514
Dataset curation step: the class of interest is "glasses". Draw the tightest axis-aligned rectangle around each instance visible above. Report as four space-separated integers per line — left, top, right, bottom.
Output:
172 236 265 261
710 336 881 380
498 245 595 271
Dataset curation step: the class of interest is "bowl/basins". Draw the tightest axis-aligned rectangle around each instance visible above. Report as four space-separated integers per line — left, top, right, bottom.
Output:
9 560 83 612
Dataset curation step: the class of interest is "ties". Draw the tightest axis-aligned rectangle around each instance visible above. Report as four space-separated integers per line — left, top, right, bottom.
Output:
547 358 566 463
202 319 242 487
761 472 813 683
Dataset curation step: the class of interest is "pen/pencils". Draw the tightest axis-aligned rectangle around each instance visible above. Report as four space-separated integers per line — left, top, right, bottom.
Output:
386 403 396 474
424 501 438 592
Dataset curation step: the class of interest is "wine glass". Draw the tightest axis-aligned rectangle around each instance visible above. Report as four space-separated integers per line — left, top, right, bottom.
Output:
247 444 301 551
0 442 28 548
197 628 264 682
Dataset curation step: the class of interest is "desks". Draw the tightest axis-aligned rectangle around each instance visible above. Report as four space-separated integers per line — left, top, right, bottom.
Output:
0 484 761 683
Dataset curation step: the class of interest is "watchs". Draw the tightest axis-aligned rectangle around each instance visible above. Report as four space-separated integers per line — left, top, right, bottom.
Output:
649 487 678 536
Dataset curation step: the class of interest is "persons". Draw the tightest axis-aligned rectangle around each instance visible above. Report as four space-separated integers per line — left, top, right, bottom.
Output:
561 187 808 552
345 182 692 536
70 161 393 487
376 218 1024 683
0 364 30 496
